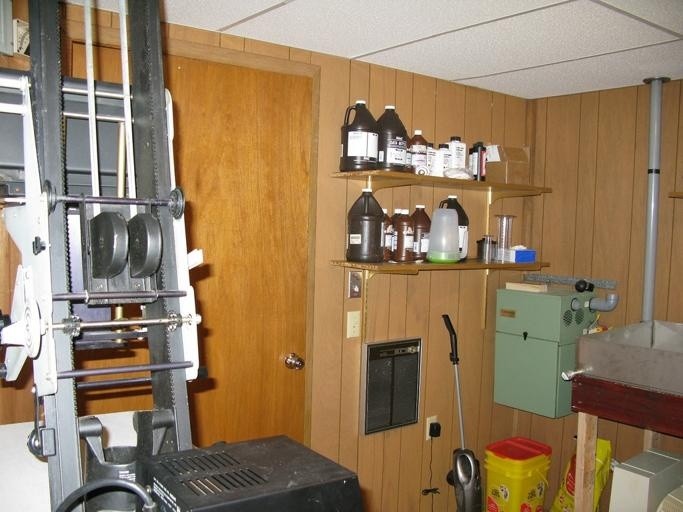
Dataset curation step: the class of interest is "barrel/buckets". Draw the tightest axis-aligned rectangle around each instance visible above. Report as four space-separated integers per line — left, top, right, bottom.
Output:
344 190 383 263
439 196 470 262
482 438 552 511
376 105 408 170
339 100 379 171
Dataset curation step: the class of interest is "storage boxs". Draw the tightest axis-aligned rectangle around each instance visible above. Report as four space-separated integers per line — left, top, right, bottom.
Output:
485 143 532 186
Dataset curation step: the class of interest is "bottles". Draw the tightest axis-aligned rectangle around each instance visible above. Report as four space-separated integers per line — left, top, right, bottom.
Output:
379 203 461 265
404 129 487 182
482 215 512 265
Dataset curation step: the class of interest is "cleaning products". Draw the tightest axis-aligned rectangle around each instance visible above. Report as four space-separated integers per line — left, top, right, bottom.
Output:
407 129 487 181
383 207 432 263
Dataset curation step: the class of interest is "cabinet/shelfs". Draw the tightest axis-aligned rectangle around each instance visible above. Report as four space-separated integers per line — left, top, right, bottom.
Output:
331 166 554 279
